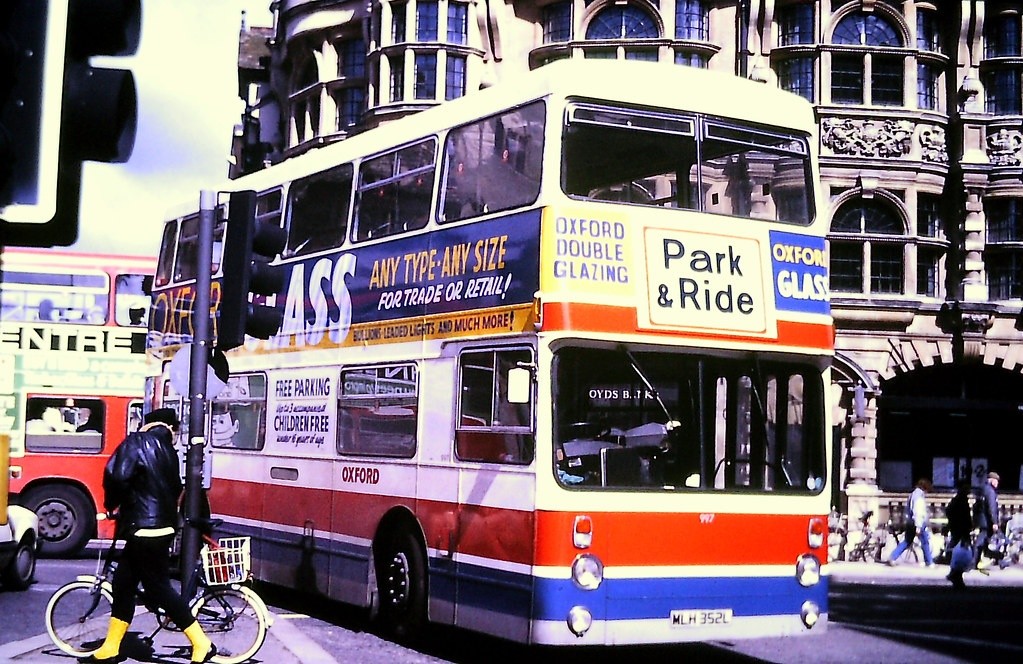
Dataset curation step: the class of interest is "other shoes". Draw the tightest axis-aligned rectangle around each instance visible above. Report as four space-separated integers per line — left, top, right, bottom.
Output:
887 558 895 566
190 642 217 664
926 563 939 569
946 569 966 587
77 654 123 664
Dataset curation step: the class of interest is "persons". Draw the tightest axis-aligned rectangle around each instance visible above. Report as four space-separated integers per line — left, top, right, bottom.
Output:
946 476 971 589
887 479 938 567
497 395 534 464
75 407 218 664
971 472 1011 571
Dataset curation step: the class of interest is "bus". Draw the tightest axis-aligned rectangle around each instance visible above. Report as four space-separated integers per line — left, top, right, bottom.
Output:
0 246 160 555
125 62 829 648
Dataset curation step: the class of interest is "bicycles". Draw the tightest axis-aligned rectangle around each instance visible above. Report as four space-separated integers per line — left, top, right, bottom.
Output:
45 510 274 664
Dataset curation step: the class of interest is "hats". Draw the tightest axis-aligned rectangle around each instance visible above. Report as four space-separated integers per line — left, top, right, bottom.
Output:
140 408 183 427
987 472 1000 480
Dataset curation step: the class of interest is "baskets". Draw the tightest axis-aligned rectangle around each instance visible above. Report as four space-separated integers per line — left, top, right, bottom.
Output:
200 536 252 587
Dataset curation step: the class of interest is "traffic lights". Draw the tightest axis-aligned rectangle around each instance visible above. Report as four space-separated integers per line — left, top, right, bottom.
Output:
61 0 140 162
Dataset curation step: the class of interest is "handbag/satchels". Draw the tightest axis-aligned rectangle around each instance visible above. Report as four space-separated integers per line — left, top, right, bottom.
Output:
951 540 974 573
912 533 924 547
983 530 1007 560
